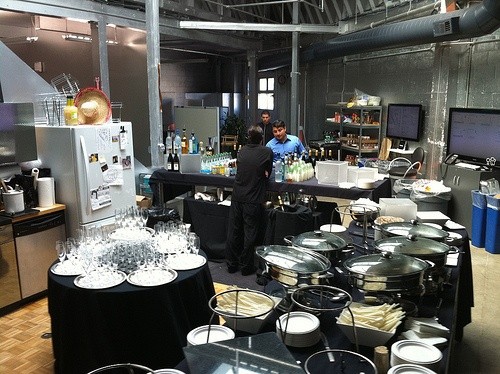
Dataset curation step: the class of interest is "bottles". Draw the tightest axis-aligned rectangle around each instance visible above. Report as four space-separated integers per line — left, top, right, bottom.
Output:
274 148 332 183
64 96 78 126
164 128 242 177
321 111 380 150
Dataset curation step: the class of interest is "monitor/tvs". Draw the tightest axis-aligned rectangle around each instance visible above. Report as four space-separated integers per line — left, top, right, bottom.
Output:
385 103 424 150
447 108 500 171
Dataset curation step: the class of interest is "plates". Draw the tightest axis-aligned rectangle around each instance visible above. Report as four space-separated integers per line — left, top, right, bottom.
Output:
50 260 85 276
107 226 155 241
276 311 321 347
164 254 207 271
186 325 236 347
73 270 126 289
388 340 443 374
127 267 178 288
339 166 378 189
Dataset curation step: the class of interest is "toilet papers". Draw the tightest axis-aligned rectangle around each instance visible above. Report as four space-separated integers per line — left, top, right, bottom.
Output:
359 95 380 105
36 178 55 207
31 168 39 190
373 102 379 106
357 100 367 105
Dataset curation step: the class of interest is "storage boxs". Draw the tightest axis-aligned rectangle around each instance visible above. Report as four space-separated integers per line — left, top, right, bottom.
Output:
136 194 151 209
379 197 417 222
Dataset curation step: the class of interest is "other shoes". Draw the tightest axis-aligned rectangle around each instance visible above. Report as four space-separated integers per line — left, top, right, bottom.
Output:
241 268 257 275
229 267 237 273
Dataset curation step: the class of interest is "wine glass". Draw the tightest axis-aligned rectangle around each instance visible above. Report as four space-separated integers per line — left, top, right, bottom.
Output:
55 205 200 289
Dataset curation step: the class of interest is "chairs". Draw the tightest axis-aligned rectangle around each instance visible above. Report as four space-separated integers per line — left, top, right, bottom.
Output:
387 147 424 196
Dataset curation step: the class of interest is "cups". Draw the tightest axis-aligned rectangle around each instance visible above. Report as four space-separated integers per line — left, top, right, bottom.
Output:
3 190 24 213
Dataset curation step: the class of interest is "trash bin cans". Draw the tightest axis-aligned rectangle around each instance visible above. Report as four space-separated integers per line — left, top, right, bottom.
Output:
389 166 417 198
144 207 179 229
396 178 451 216
471 189 500 255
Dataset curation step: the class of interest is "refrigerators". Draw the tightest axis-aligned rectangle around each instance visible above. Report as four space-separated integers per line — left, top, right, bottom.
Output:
36 121 138 239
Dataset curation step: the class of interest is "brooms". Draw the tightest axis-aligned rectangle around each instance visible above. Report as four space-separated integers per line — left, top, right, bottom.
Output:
303 130 309 148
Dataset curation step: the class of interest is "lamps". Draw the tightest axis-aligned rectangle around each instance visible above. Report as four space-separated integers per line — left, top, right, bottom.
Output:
61 18 119 45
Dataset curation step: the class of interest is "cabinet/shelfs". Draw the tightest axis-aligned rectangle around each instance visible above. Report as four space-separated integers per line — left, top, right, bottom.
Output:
340 104 382 162
437 162 500 242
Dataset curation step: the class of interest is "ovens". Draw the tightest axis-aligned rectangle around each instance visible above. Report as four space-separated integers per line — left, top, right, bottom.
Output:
13 211 69 302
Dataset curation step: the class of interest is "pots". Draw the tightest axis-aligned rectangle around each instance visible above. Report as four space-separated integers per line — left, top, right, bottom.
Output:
253 218 459 305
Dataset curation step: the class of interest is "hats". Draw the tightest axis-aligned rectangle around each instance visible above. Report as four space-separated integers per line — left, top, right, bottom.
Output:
73 89 111 125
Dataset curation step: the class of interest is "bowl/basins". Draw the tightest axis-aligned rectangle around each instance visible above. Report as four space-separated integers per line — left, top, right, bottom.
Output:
334 300 397 347
214 287 282 335
416 210 450 225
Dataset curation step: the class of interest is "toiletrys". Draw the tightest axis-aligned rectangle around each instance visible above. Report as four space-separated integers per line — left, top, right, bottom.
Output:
325 131 331 143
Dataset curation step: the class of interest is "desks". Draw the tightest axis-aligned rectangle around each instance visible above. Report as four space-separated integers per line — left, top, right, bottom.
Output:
183 189 341 260
46 257 220 374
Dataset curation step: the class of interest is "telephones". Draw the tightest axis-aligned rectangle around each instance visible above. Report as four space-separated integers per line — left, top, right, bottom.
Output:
444 152 459 165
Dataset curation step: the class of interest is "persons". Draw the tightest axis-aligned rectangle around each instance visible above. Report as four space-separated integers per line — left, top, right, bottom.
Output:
255 110 274 145
265 120 309 163
226 126 274 276
